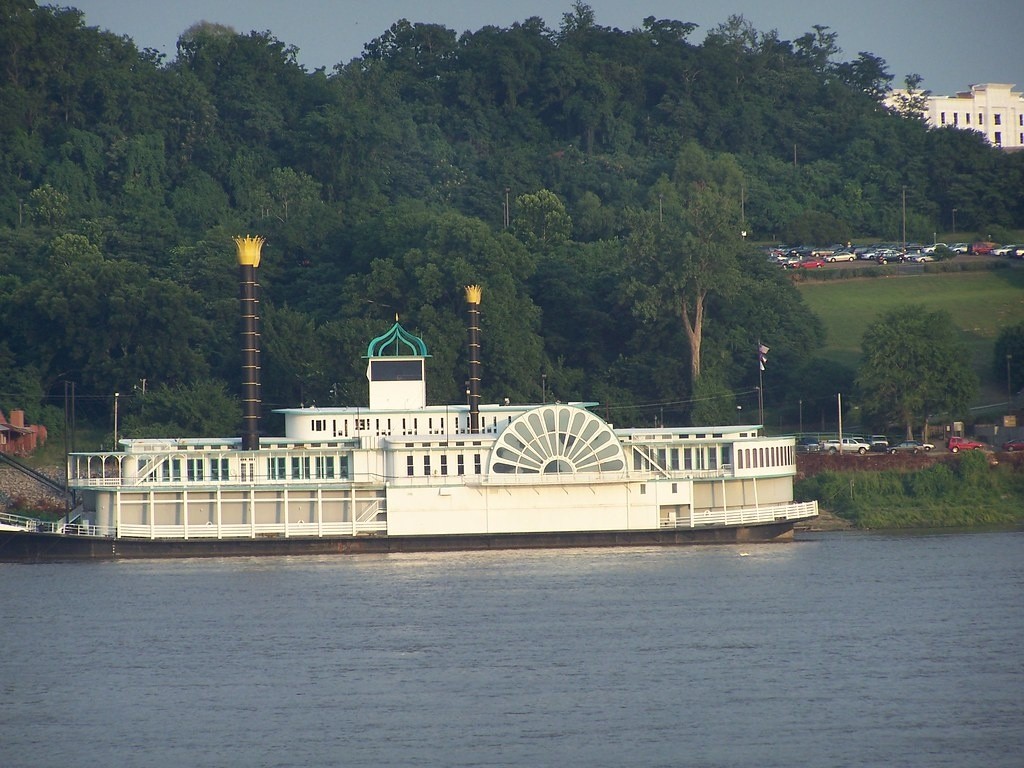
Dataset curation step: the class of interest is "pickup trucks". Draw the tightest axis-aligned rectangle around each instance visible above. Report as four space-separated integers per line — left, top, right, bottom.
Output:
948 436 989 453
823 438 870 455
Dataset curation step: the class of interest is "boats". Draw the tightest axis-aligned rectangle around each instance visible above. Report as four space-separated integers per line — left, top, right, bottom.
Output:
0 233 823 568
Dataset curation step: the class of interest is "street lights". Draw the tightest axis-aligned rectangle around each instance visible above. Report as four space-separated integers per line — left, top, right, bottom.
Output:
113 392 119 466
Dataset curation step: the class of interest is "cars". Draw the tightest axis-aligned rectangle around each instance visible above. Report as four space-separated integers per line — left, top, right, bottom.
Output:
1002 439 1024 451
805 434 936 455
769 238 1023 271
